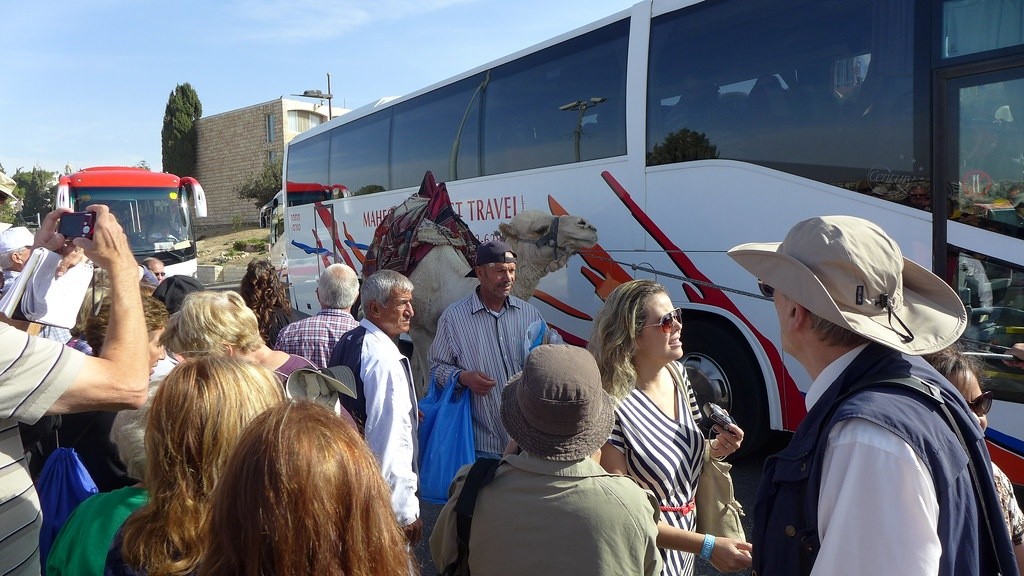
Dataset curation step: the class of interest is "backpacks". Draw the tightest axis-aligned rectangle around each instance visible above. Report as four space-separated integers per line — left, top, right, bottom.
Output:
35 413 99 576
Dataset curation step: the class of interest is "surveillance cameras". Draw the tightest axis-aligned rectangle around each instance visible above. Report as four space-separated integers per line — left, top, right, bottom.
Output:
559 101 583 111
591 98 608 104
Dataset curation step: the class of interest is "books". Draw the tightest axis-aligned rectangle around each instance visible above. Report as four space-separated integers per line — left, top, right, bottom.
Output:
0 248 94 330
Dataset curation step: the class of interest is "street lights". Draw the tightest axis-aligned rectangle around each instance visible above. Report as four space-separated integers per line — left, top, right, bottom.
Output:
558 97 607 162
327 72 331 121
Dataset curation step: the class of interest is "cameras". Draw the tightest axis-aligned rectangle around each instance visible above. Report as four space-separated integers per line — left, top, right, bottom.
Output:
703 401 737 435
58 213 96 240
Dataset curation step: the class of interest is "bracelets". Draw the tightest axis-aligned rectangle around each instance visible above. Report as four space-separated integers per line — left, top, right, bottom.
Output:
699 534 715 562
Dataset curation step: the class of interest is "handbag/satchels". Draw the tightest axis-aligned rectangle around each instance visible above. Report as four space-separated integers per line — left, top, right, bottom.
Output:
416 365 476 504
436 458 495 576
695 457 752 559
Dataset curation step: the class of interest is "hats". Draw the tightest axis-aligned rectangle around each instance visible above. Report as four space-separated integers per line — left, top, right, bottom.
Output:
726 215 968 355
500 344 615 462
0 227 34 253
465 240 517 278
285 365 357 410
152 275 205 314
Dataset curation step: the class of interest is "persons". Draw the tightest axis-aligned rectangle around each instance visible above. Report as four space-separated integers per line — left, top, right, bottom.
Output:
587 279 752 576
0 167 150 576
40 258 421 576
728 215 1024 576
427 240 549 469
428 343 663 576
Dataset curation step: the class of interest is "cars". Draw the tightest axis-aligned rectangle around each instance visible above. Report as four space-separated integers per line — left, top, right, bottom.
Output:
964 305 1024 402
961 197 1021 302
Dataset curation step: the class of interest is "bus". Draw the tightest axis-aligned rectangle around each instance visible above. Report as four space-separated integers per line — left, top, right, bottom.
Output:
259 191 288 284
284 1 1024 513
54 164 208 285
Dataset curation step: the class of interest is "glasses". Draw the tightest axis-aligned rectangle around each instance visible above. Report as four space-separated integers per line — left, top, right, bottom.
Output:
643 308 682 333
965 391 992 416
757 279 774 298
155 273 165 276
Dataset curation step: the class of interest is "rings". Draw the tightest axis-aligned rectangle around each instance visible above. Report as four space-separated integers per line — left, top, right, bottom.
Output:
724 442 731 450
